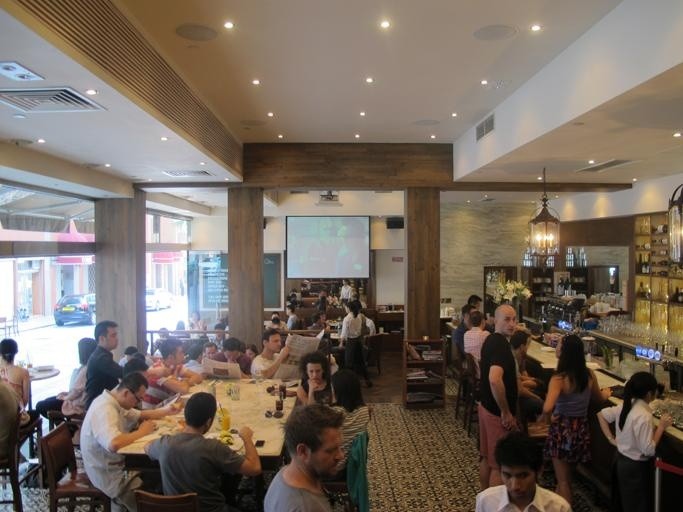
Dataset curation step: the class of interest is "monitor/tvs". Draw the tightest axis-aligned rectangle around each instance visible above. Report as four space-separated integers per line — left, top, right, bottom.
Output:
590 264 621 295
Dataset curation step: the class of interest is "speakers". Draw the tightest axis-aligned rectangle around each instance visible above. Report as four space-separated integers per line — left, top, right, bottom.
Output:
387 218 404 228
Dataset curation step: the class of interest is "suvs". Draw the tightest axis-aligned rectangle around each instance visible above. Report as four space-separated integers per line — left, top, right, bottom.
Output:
53 293 96 326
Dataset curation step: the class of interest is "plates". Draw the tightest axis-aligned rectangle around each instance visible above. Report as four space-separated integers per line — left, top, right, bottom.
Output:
203 433 244 451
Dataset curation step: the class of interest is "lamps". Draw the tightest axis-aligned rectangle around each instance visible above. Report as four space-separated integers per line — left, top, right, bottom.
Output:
668 184 683 271
528 167 562 273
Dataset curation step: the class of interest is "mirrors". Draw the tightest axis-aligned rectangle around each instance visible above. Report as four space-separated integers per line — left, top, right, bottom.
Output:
561 241 631 313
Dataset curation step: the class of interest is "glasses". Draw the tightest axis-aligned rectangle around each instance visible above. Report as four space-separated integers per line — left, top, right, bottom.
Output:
128 386 145 402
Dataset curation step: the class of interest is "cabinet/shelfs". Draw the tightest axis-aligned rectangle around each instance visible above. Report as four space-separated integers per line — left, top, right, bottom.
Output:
364 333 390 376
402 337 446 409
633 212 683 342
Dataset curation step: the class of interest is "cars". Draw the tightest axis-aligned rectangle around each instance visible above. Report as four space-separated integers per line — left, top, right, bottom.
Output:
145 288 171 311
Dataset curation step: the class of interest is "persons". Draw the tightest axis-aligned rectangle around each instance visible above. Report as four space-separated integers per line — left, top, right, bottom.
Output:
0 338 30 427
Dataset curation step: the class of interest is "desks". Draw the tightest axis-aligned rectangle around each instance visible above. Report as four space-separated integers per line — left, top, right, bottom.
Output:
114 378 302 505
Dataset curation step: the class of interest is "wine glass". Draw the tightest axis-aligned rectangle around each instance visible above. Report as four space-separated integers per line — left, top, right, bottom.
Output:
254 365 265 398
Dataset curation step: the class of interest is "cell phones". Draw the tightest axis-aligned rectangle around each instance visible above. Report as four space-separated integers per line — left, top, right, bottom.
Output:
256 441 264 446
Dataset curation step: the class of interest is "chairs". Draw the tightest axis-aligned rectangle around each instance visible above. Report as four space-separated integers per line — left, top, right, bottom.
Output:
455 341 466 418
37 421 111 511
1 409 65 511
131 489 207 511
319 431 368 511
463 354 480 437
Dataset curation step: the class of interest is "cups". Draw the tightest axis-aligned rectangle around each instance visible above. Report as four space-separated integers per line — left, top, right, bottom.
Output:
213 407 230 430
598 314 681 358
650 395 682 427
231 383 240 402
274 381 287 411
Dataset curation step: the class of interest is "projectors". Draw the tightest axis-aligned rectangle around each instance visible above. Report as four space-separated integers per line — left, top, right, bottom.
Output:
319 195 340 203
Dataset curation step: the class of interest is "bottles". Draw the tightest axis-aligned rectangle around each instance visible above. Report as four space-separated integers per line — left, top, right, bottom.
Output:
556 273 572 296
636 222 682 302
